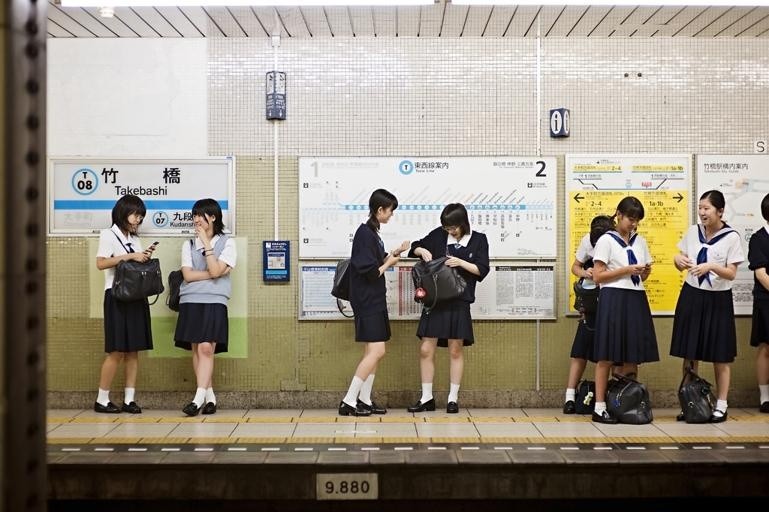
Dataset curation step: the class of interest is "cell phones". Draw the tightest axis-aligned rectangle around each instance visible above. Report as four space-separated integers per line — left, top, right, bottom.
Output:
642 261 656 267
144 241 160 252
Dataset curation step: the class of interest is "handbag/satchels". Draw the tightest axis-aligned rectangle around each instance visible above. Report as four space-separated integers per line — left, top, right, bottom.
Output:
678 361 717 423
113 257 164 304
576 379 596 412
331 260 356 319
573 269 602 318
169 268 184 309
411 257 466 311
605 372 654 424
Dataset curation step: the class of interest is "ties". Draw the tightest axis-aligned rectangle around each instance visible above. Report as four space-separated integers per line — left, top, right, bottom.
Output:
455 243 461 250
202 249 207 258
126 242 138 255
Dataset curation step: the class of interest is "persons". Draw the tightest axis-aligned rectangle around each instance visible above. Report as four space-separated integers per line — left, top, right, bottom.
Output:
591 196 660 423
563 216 617 412
340 189 410 416
748 192 769 412
674 190 743 422
408 203 489 414
92 196 153 413
175 198 238 415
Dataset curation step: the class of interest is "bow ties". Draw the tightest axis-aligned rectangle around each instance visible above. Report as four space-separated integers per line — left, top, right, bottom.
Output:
611 234 641 286
698 225 730 286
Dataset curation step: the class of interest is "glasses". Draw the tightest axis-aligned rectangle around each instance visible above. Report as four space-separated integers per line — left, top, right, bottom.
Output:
443 224 460 232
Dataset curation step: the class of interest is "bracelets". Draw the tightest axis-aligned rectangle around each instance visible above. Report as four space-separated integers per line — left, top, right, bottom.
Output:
201 248 214 257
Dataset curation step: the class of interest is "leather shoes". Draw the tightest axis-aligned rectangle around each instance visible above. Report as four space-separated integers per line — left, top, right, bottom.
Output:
760 400 769 414
183 401 202 416
340 399 372 415
203 401 219 414
592 410 619 423
711 407 727 422
358 400 387 413
408 397 437 412
121 399 142 413
95 401 120 413
447 401 458 413
564 400 576 414
